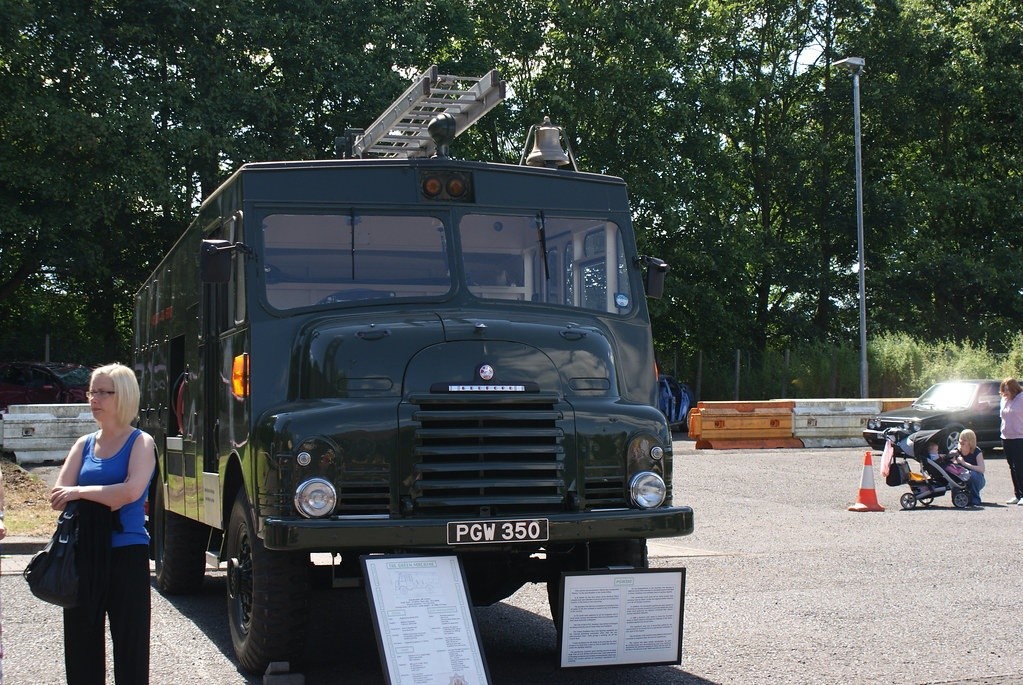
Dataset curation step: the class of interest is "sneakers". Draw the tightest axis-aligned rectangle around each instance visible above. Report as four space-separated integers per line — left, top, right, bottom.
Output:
1017 498 1023 505
1006 496 1019 504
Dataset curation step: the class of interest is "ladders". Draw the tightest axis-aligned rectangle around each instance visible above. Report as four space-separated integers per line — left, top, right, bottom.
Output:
354 64 508 164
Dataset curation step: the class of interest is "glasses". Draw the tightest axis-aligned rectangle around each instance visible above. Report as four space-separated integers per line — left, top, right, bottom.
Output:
85 391 115 399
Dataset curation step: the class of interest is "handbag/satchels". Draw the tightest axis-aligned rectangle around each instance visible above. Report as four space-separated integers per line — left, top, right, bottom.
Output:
24 500 79 609
886 444 910 486
881 440 894 477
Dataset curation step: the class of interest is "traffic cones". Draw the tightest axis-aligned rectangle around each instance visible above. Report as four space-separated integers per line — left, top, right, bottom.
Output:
847 451 886 512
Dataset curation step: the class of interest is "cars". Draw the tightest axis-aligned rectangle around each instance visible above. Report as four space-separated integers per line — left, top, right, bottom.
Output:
0 360 97 423
862 378 1023 459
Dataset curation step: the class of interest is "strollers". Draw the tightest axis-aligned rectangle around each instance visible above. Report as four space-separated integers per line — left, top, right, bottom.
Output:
882 422 973 511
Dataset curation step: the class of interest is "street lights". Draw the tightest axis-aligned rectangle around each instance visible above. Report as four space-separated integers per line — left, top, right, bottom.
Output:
831 56 869 399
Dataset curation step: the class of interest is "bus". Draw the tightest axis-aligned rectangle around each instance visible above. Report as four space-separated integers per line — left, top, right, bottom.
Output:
130 64 696 677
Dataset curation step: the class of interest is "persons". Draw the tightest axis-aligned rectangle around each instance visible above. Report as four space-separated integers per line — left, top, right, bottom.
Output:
51 363 155 684
0 461 6 546
926 441 971 484
947 429 986 506
997 377 1023 507
